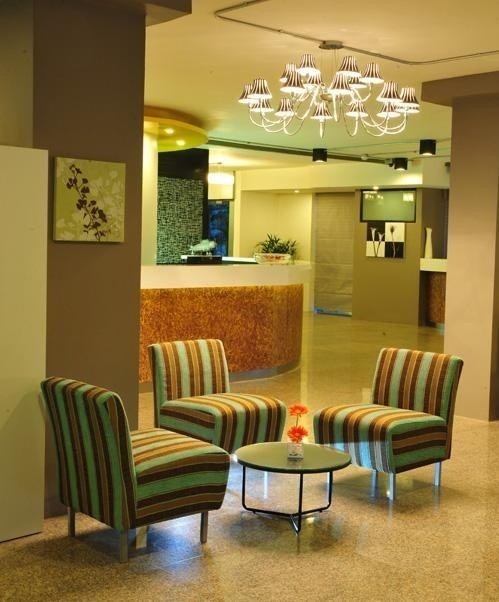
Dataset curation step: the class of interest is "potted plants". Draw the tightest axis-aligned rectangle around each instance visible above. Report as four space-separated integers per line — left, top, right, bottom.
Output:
253 234 297 264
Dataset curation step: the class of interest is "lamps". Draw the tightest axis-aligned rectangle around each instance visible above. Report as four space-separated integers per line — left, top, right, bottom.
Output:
394 158 408 171
238 41 421 141
419 138 437 156
312 148 328 163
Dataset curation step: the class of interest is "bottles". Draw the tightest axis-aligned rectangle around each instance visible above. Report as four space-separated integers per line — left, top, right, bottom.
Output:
424 228 433 260
